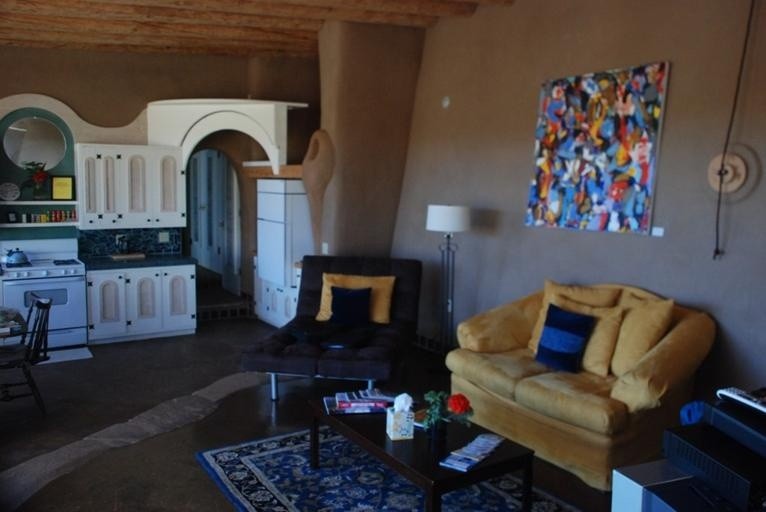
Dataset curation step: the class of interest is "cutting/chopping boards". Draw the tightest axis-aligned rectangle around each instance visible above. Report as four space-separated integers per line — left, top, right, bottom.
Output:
110 253 147 261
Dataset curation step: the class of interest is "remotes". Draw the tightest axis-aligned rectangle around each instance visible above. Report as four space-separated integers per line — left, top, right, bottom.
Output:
716 386 766 416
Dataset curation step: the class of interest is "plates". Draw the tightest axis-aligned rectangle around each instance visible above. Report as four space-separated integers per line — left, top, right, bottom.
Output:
0 183 20 201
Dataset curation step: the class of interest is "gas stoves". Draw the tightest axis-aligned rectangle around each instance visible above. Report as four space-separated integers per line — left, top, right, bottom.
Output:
1 259 85 279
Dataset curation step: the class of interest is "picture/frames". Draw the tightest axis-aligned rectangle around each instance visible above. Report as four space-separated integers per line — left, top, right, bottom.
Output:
49 174 75 202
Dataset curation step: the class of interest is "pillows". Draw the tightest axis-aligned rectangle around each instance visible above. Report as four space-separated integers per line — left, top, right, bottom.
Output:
535 303 599 374
526 279 621 357
610 291 675 378
534 293 623 377
328 285 375 327
312 271 396 327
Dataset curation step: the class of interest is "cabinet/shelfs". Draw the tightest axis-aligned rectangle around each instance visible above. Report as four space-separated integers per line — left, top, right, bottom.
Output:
74 142 127 229
127 144 188 229
126 264 196 341
248 255 305 328
253 177 310 284
0 199 77 228
86 268 127 341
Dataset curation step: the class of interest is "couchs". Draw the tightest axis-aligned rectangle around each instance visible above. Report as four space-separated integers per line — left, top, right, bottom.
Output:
446 282 720 494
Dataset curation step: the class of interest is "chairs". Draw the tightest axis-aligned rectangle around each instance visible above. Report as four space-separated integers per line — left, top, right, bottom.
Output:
240 251 423 403
0 293 54 415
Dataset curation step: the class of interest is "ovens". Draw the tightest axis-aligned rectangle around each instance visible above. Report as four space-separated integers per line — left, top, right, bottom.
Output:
2 277 88 351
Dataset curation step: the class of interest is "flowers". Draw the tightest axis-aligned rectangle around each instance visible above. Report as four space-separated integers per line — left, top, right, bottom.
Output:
19 160 48 189
421 392 474 428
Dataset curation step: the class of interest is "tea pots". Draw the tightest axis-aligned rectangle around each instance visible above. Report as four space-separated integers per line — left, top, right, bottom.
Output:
5 247 28 264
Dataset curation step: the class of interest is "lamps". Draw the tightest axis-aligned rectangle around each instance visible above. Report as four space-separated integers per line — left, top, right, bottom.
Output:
425 202 471 360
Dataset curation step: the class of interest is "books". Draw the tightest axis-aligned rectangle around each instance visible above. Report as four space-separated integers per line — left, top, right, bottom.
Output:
322 388 391 414
438 431 502 473
19 208 78 224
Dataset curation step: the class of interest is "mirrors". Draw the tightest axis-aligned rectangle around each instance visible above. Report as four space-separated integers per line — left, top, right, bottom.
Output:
0 107 74 174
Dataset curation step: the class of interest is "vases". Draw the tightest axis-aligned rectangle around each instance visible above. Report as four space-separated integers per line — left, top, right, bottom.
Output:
427 420 450 456
32 183 48 200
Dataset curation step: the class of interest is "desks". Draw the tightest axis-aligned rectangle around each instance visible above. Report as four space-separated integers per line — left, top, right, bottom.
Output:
0 305 27 340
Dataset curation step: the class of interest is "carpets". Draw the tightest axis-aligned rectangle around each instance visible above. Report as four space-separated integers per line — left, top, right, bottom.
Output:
191 423 585 511
32 345 93 366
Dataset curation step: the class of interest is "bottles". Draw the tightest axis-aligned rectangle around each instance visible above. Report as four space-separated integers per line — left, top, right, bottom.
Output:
7 210 76 223
